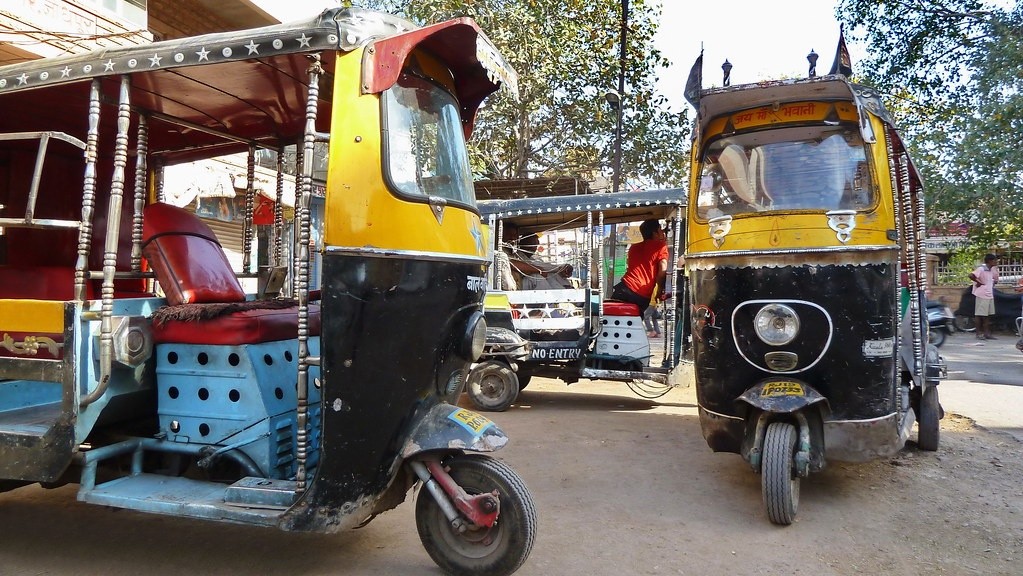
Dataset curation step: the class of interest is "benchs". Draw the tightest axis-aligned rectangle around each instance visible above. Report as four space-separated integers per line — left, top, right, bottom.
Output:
1 263 94 332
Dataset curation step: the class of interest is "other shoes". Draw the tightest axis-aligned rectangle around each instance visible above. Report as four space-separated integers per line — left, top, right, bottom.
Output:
655 333 661 338
976 334 985 340
648 331 657 338
984 334 996 339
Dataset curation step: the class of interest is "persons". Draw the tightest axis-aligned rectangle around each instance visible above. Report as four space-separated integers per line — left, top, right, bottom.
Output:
510 232 574 290
644 283 672 337
611 218 669 321
968 254 999 340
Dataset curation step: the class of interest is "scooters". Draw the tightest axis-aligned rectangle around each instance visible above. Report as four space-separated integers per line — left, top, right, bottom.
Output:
951 284 1023 333
925 299 957 348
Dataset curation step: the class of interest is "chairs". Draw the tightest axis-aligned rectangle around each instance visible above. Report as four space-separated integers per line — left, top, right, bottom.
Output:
585 256 640 315
140 202 321 345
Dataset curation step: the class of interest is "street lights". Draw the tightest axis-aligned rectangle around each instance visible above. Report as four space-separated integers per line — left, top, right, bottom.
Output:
601 0 630 297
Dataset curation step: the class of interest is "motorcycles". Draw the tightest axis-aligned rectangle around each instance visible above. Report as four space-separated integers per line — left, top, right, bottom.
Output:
0 6 539 576
682 76 946 526
467 185 695 413
